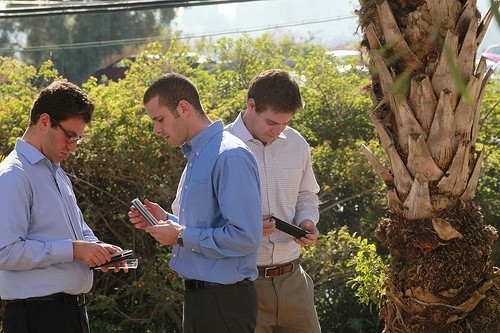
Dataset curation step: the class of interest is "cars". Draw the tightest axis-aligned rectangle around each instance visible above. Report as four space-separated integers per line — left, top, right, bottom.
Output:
90 49 372 85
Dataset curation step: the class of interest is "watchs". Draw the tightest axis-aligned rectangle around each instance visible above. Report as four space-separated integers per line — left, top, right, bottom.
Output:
177 228 184 247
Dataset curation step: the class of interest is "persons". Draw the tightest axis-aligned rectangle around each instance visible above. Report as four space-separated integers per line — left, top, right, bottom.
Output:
0 81 128 333
224 69 323 333
128 74 264 333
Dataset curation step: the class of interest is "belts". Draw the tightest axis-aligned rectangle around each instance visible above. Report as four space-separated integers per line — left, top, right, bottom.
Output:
6 291 88 308
181 277 219 293
257 257 302 279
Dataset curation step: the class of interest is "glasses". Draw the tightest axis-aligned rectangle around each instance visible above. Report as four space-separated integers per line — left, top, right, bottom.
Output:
51 117 84 144
